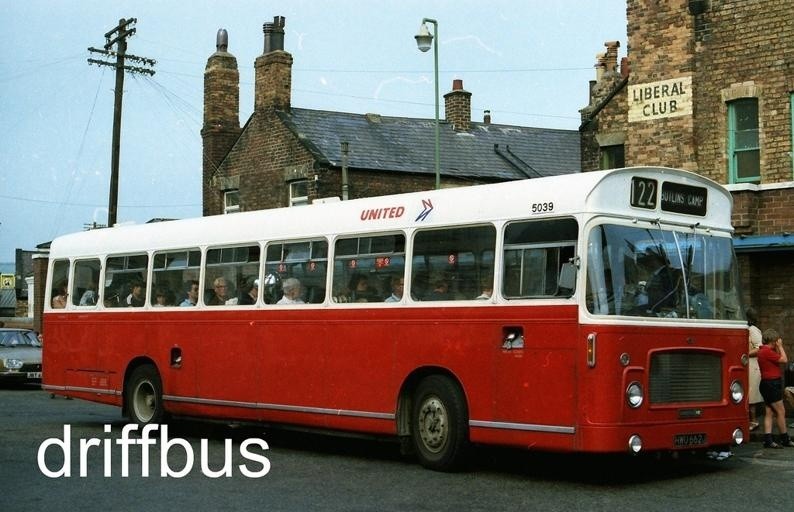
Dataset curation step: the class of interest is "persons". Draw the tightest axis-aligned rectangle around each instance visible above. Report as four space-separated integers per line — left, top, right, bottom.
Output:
383 257 391 266
448 254 457 265
280 264 286 274
350 259 357 269
744 303 764 433
53 271 509 310
310 262 316 272
638 245 690 314
758 327 794 449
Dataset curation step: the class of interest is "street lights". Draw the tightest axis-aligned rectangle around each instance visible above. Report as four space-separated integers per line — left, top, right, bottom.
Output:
414 18 441 190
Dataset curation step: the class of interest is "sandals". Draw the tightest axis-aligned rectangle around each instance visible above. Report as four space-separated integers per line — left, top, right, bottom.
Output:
749 421 759 431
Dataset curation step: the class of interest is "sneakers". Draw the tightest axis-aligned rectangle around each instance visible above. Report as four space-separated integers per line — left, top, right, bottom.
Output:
763 439 794 448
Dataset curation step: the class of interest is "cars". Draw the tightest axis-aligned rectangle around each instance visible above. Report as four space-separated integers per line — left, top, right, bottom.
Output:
0 327 43 383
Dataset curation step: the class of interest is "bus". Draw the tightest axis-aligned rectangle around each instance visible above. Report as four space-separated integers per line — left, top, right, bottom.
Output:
41 165 750 470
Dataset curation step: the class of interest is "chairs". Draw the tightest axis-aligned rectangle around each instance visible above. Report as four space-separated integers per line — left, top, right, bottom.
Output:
50 278 477 308
